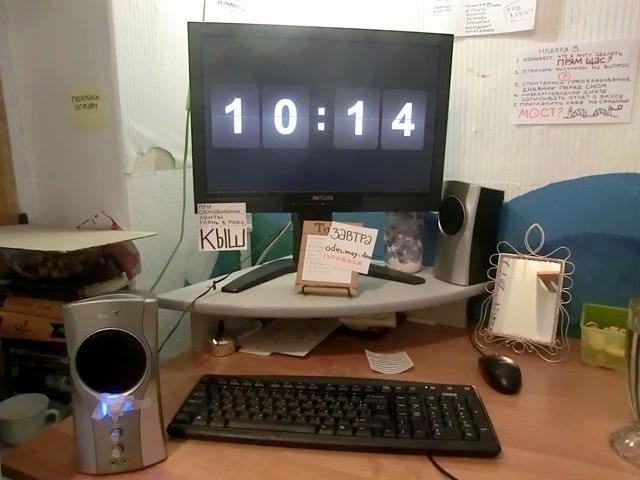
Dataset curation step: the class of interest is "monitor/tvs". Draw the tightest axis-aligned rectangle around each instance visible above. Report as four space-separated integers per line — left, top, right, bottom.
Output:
187 20 454 293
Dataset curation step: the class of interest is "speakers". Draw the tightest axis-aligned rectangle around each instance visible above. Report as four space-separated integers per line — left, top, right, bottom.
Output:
60 291 168 476
433 177 505 286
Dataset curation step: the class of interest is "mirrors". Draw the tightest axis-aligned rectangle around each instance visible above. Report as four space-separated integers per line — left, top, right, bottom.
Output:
471 221 577 365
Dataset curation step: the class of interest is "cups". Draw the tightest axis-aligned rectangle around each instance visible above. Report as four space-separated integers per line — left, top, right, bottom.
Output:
0 393 59 446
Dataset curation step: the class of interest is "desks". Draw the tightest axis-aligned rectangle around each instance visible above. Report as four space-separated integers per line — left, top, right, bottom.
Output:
1 250 640 480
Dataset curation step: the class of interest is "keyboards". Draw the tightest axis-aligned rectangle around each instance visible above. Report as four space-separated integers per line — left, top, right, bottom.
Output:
165 373 502 459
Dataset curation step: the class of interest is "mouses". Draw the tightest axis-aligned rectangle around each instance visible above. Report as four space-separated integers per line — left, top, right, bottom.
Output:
478 353 522 395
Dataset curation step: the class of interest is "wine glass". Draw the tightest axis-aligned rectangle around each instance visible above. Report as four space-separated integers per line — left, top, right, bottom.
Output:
609 297 640 467
